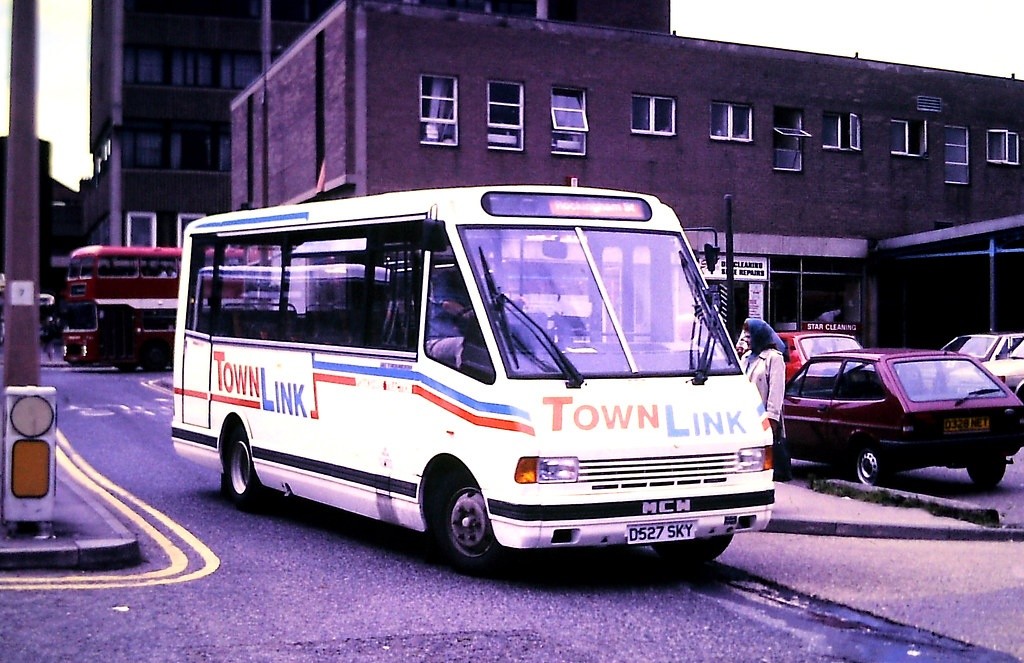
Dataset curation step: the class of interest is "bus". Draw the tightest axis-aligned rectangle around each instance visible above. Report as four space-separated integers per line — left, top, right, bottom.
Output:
59 244 275 374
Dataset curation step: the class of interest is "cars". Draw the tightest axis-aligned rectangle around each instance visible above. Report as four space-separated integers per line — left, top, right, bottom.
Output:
778 347 1024 490
934 333 1024 401
775 331 867 397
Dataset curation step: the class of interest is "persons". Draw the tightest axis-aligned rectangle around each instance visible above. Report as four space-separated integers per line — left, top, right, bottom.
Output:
741 319 788 428
426 272 474 370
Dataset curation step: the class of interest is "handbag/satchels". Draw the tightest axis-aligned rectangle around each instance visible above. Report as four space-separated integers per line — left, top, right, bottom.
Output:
770 414 793 482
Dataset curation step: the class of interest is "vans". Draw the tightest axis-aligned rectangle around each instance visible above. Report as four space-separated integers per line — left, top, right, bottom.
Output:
170 185 779 581
